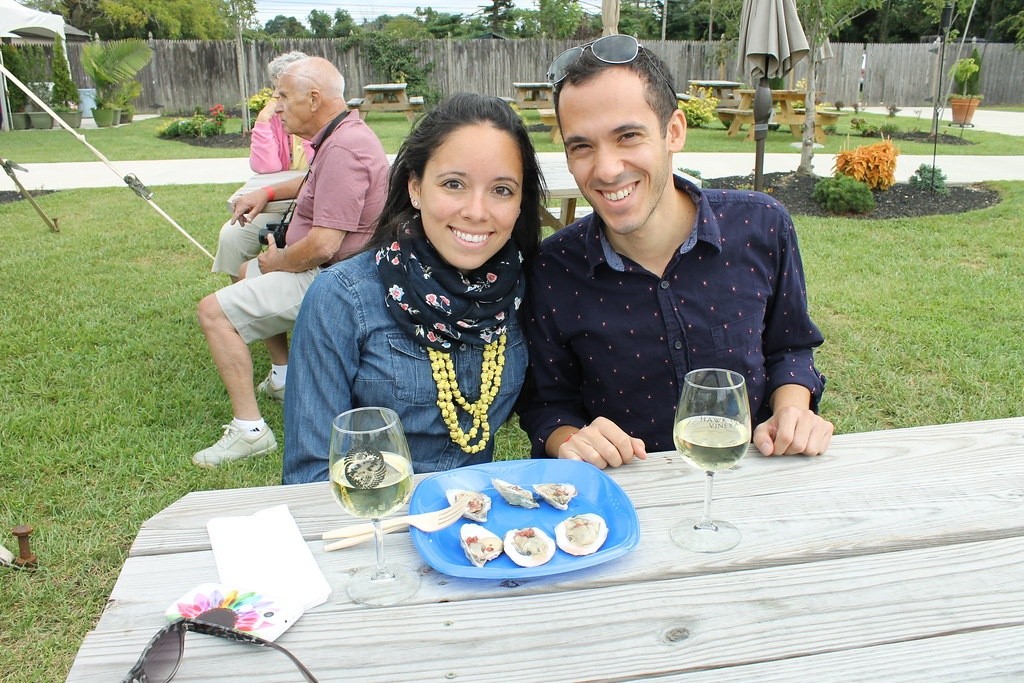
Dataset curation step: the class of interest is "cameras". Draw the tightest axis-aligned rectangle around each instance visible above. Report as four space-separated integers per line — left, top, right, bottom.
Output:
258 220 289 248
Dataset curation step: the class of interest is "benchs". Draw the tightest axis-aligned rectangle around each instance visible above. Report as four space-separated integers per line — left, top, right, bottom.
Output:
542 203 596 220
500 96 516 103
409 95 424 105
538 106 559 127
347 97 363 107
717 105 754 117
794 105 850 117
676 92 695 101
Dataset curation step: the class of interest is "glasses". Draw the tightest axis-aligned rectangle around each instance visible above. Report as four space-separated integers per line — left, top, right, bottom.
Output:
277 66 320 86
546 33 679 111
122 616 319 683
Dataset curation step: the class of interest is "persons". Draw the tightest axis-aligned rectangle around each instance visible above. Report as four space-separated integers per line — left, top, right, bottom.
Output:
249 52 315 171
193 56 390 465
512 35 834 469
283 92 547 484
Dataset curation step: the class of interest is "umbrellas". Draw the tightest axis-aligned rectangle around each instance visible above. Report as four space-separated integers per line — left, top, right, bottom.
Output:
601 0 620 38
735 0 810 81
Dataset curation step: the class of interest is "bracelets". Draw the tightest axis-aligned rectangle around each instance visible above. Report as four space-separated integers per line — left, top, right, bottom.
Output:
262 186 274 201
566 432 577 442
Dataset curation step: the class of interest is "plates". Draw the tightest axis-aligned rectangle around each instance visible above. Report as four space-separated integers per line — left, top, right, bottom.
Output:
409 459 641 580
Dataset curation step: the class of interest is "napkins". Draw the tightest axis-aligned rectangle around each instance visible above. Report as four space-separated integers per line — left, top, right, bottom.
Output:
206 505 331 612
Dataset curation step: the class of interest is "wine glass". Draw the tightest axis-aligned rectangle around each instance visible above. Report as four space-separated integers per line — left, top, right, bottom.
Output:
328 406 421 607
669 368 753 552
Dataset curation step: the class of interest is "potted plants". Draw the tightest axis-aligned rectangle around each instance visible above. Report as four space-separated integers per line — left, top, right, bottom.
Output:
946 58 984 122
1 29 153 130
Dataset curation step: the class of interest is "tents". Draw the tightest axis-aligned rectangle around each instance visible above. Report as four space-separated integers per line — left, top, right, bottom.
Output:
0 0 93 128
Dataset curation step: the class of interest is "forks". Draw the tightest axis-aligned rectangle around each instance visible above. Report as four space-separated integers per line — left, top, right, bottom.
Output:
321 493 473 551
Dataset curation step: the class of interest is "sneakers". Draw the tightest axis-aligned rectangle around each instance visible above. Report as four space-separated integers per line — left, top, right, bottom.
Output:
192 420 277 469
256 369 285 404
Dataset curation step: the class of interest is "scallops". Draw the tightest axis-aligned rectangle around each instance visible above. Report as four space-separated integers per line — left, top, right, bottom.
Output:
503 525 556 568
554 512 609 556
445 488 492 523
490 477 540 509
460 523 502 568
532 482 576 509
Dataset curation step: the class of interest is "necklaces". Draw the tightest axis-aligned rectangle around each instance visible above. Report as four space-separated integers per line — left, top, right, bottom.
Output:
427 334 506 452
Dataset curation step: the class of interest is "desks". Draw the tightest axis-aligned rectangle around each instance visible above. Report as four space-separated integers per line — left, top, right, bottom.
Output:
513 81 555 109
222 151 704 235
66 414 1024 683
686 79 745 133
360 82 415 131
732 87 827 144
